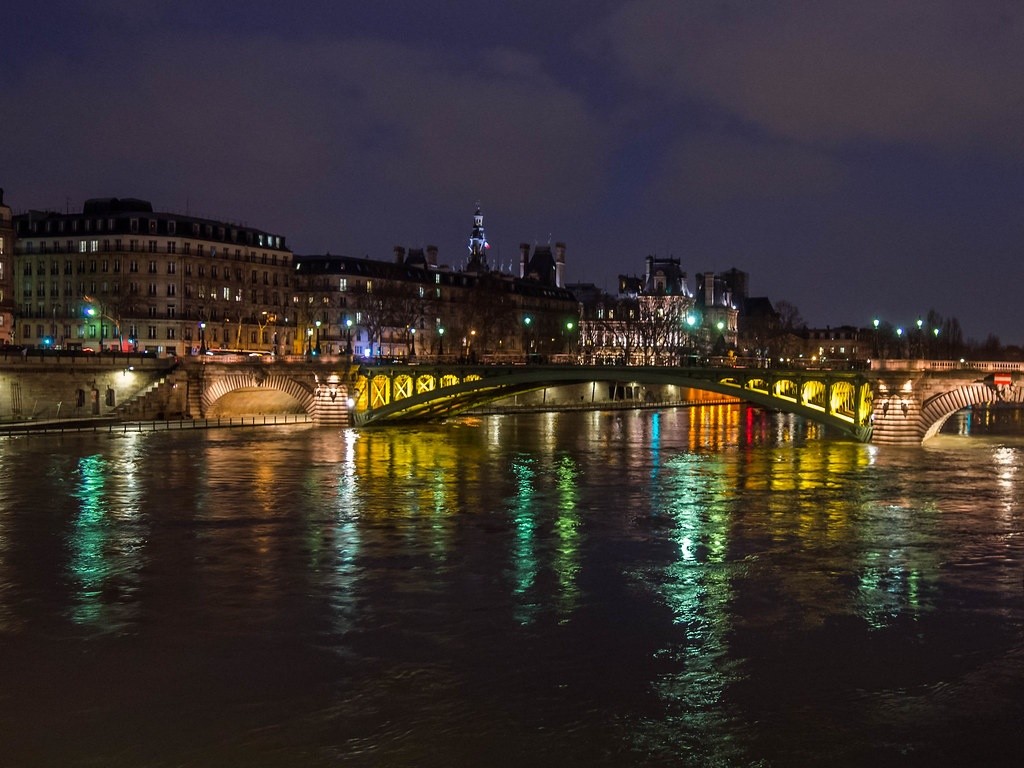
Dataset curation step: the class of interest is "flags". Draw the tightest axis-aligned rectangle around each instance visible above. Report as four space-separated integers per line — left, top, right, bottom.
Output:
485 242 490 249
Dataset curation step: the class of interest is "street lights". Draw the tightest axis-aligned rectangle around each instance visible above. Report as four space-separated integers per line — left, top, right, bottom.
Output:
687 315 693 375
410 328 416 355
471 330 476 357
873 319 881 359
716 321 724 357
201 322 206 354
88 295 103 353
897 328 902 359
261 311 277 356
933 328 939 357
567 322 573 363
439 327 444 355
917 316 922 356
129 336 136 354
524 317 530 364
346 319 354 355
316 319 322 354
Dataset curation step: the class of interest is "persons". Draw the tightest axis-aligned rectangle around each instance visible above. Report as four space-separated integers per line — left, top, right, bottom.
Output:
616 351 624 365
270 346 277 355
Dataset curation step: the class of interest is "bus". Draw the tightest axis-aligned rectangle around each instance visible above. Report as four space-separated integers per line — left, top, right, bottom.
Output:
207 349 271 356
